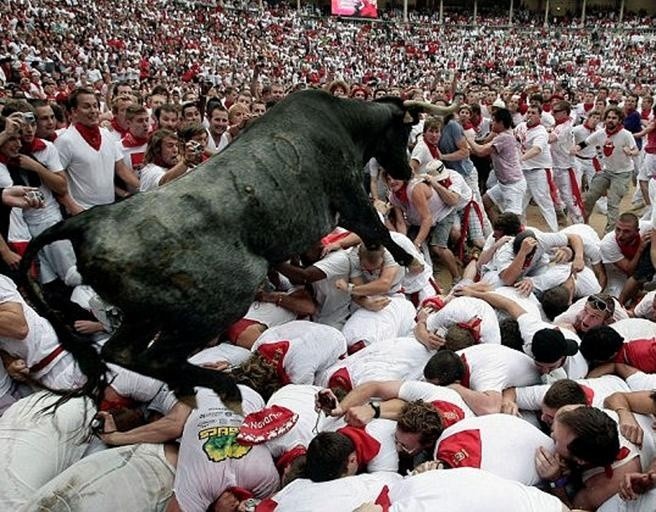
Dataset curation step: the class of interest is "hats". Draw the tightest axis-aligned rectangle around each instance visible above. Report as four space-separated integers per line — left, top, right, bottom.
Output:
426 160 448 181
531 328 578 356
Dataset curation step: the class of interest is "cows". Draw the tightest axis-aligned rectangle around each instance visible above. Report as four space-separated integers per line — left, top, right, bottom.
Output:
16 88 462 444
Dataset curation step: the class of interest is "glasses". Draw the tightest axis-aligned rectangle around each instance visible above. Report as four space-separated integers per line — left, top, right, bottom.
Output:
587 294 612 313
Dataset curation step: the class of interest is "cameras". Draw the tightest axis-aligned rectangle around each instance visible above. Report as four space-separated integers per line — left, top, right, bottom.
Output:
20 112 35 124
193 144 202 153
89 415 106 432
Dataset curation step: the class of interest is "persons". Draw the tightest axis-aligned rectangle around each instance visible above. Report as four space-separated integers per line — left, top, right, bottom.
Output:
2 0 656 511
